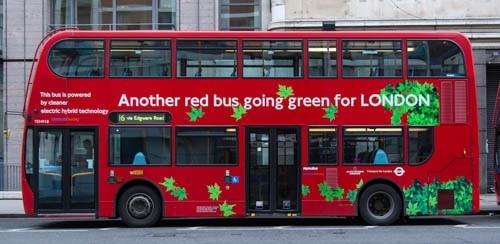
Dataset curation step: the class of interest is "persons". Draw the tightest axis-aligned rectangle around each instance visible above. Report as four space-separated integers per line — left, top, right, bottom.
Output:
83 138 93 161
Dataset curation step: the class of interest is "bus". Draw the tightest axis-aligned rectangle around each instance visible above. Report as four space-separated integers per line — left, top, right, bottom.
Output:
21 26 480 227
